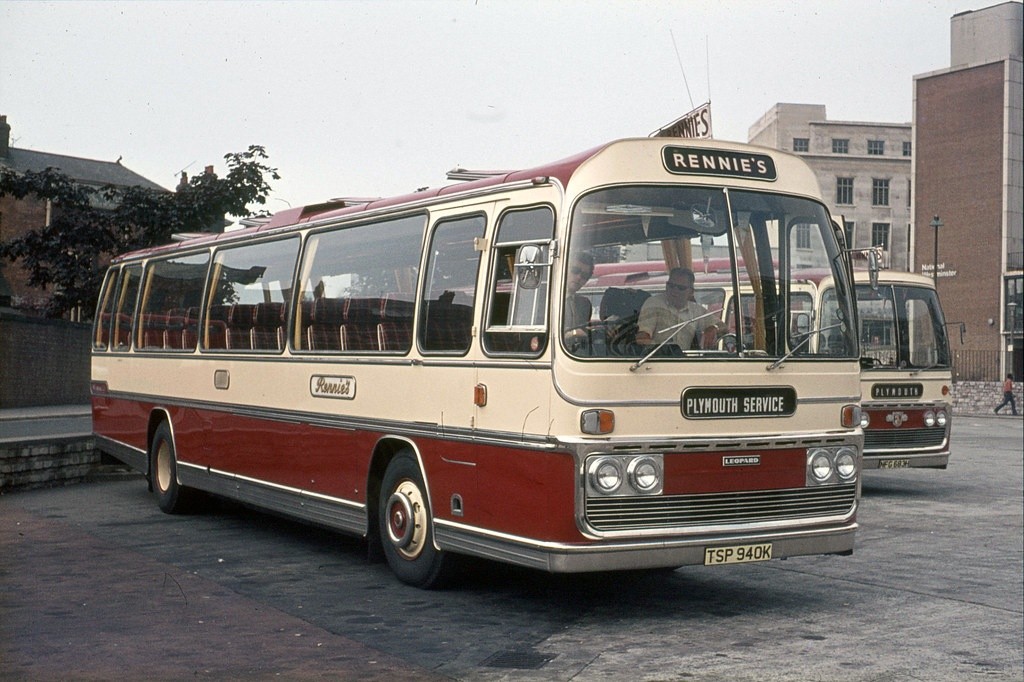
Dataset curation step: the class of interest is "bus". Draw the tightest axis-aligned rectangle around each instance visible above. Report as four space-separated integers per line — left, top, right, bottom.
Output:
89 101 891 593
433 255 967 471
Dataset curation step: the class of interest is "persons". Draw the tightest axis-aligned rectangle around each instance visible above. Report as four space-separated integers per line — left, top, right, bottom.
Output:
531 252 594 350
889 357 894 365
635 268 728 350
993 373 1017 415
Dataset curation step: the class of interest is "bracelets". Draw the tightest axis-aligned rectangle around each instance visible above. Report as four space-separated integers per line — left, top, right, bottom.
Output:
572 329 576 336
713 324 719 331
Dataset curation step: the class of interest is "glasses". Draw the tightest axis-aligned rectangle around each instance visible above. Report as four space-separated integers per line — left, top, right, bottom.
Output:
668 283 687 290
571 266 590 280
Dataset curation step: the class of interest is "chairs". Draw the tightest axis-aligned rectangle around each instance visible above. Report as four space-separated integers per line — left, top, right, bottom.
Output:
600 287 652 342
103 290 519 351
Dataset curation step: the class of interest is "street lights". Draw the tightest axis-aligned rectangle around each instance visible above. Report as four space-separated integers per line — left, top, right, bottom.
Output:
931 213 944 291
